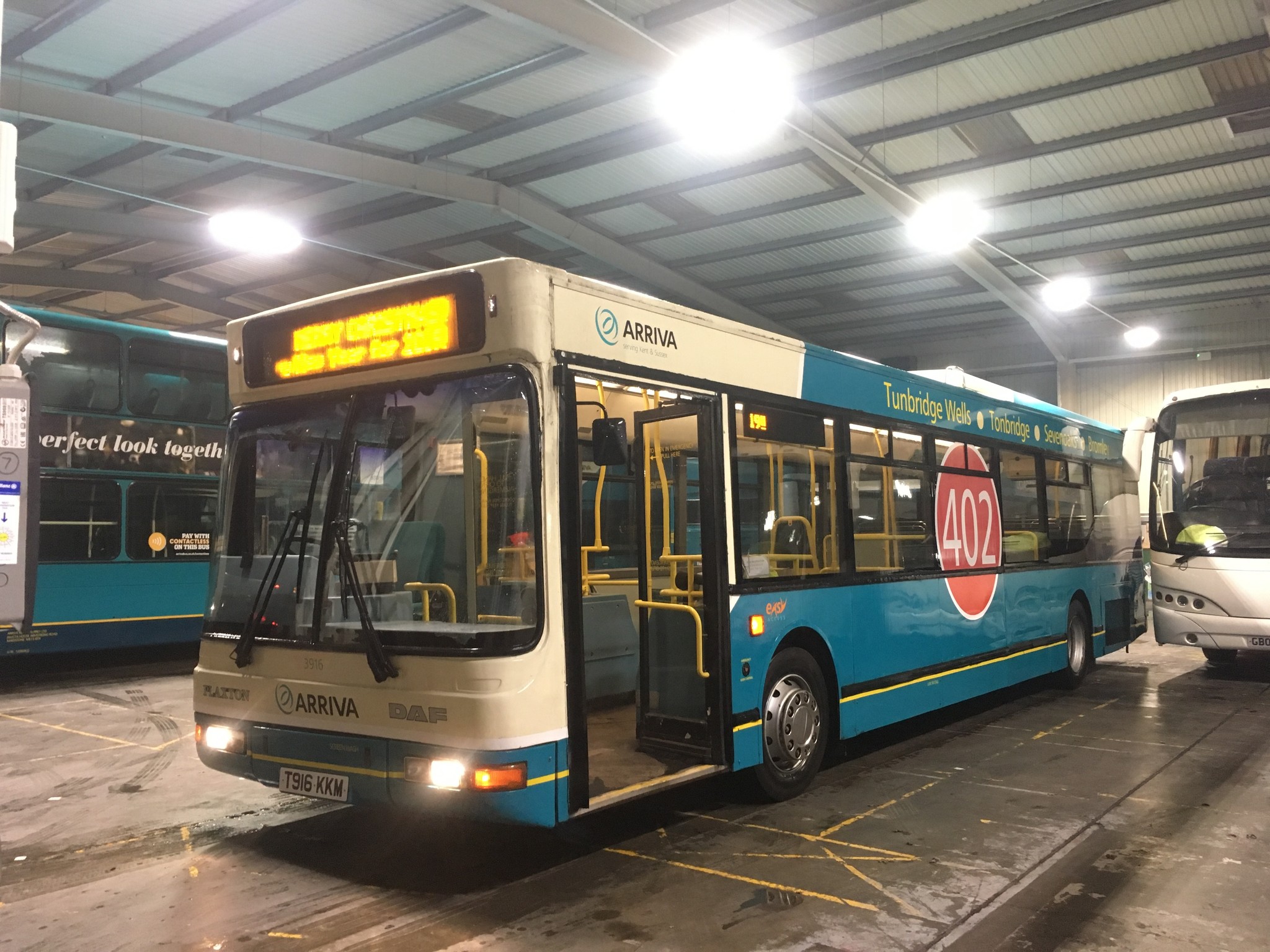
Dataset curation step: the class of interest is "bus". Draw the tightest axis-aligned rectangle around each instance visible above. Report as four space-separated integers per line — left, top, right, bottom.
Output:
195 254 1149 833
2 303 403 678
1122 378 1270 661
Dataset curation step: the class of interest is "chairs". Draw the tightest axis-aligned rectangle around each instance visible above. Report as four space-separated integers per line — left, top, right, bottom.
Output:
1201 455 1269 503
76 371 235 420
381 517 447 592
772 516 1087 571
